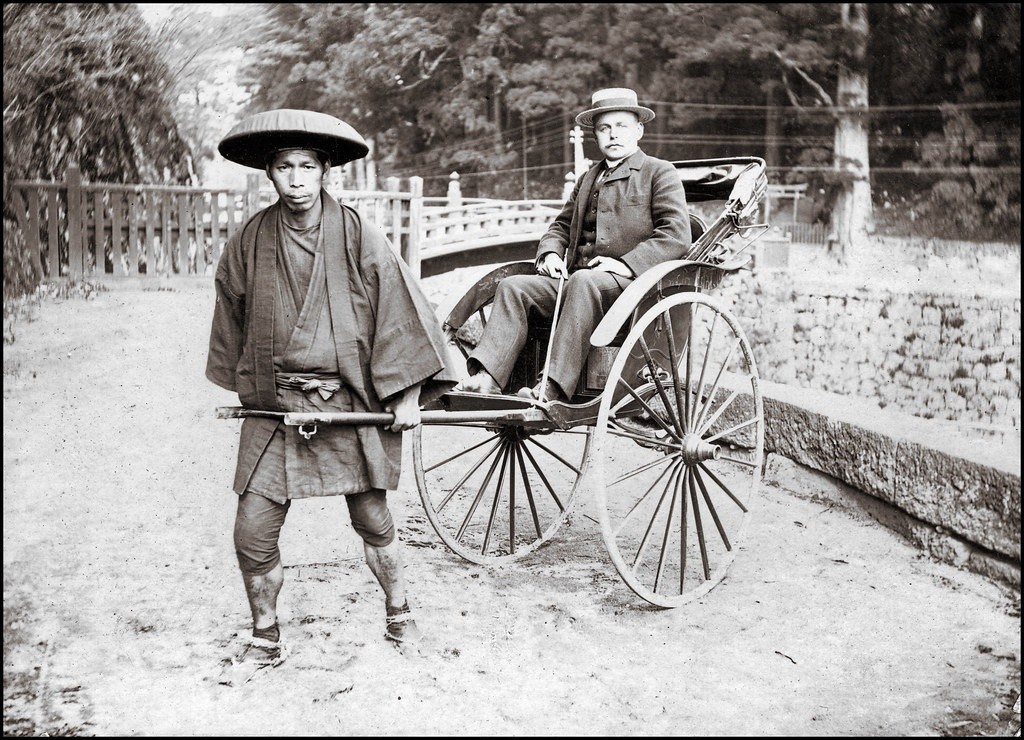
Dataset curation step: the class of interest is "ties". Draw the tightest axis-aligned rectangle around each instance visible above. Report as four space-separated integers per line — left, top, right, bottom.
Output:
600 164 620 183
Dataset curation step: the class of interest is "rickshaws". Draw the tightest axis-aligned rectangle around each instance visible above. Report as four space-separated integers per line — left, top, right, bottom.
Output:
214 155 770 609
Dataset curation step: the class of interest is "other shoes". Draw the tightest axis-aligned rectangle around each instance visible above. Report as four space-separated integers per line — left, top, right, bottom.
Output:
517 378 559 403
385 596 416 643
220 637 284 687
454 375 502 395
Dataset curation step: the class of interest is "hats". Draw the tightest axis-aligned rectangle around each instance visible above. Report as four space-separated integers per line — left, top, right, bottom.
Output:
218 108 369 170
574 88 655 127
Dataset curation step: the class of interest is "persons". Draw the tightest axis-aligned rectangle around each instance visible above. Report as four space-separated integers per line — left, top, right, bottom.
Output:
204 108 458 688
455 86 691 398
